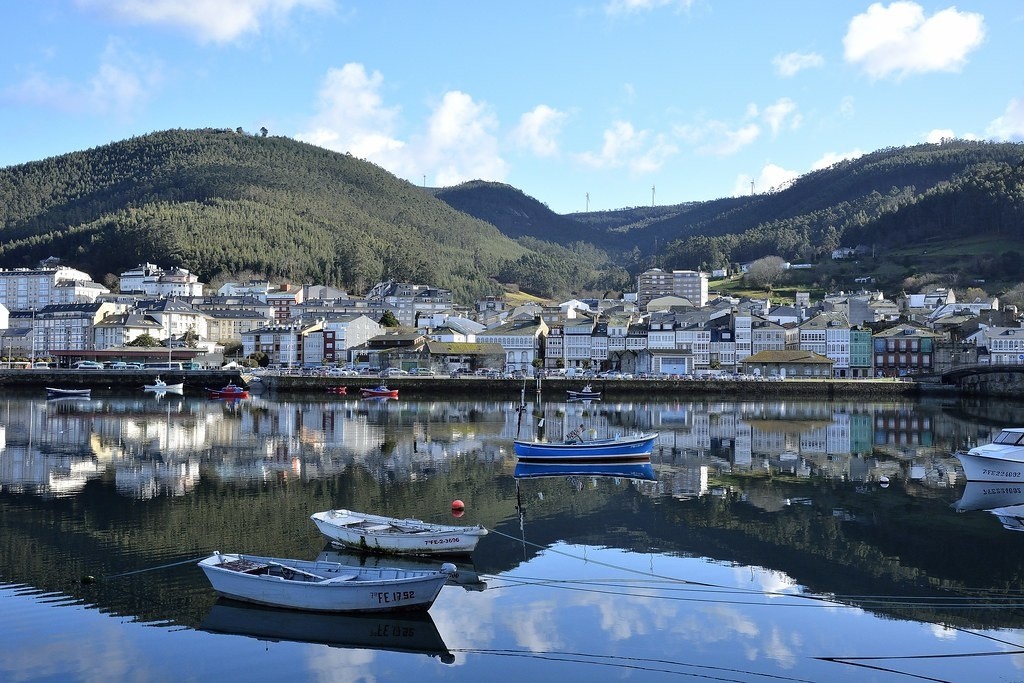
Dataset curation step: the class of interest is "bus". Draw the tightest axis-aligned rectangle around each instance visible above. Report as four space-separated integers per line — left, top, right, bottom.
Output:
139 362 183 371
0 361 32 370
179 361 202 371
33 362 59 370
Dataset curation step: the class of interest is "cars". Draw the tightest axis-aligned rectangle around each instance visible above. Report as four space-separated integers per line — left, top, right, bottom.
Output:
278 366 435 377
451 367 785 382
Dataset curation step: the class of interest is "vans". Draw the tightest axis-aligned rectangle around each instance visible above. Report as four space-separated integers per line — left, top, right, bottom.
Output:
72 360 143 372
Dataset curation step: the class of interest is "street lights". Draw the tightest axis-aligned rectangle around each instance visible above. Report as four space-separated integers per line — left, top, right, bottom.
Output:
5 337 12 370
29 308 38 370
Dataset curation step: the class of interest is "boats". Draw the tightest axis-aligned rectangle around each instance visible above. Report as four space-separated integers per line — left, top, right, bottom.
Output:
327 384 348 395
196 550 461 619
138 375 184 389
44 386 92 397
359 379 399 398
946 480 1024 538
144 389 184 404
46 397 91 403
203 381 250 401
564 383 605 405
313 541 488 592
952 428 1024 483
309 509 489 562
508 370 659 458
512 457 660 564
194 596 457 664
